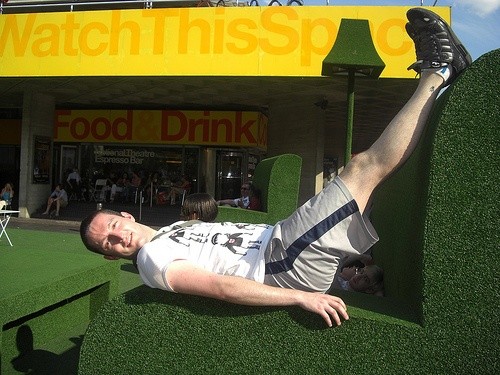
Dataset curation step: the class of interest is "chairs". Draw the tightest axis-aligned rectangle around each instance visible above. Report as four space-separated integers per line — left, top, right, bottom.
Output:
88 179 108 203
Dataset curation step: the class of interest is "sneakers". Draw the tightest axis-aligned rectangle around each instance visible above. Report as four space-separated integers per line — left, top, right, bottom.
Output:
406 7 473 87
405 22 424 72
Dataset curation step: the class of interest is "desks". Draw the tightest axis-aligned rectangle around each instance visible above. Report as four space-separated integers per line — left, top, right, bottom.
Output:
0 210 21 247
0 243 121 375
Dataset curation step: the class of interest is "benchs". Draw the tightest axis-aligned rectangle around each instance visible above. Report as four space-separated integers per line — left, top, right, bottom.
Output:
213 154 303 226
78 48 500 375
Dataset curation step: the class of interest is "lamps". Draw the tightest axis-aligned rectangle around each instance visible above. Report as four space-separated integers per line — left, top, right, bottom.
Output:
321 19 386 168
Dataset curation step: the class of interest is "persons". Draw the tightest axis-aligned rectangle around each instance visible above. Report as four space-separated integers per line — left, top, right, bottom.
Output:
197 0 213 7
80 170 198 207
41 183 68 216
182 192 218 222
81 7 472 327
331 264 384 293
0 183 14 218
215 182 262 210
67 166 81 201
